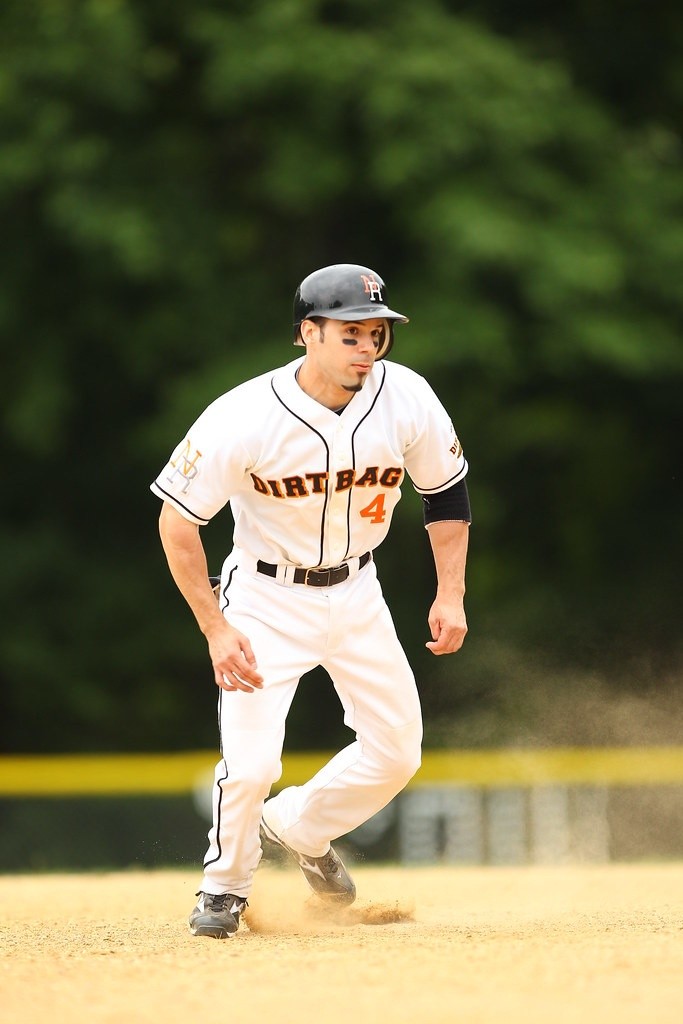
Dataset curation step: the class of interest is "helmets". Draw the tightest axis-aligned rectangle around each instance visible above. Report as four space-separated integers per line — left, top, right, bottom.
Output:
292 264 409 328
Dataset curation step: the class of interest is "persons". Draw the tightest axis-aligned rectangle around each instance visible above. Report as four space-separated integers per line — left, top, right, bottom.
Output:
151 265 472 942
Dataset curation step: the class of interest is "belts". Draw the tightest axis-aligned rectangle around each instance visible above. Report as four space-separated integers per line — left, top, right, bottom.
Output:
257 551 370 588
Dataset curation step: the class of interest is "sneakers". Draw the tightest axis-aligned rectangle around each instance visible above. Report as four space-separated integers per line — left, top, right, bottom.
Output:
186 891 249 939
260 815 357 908
